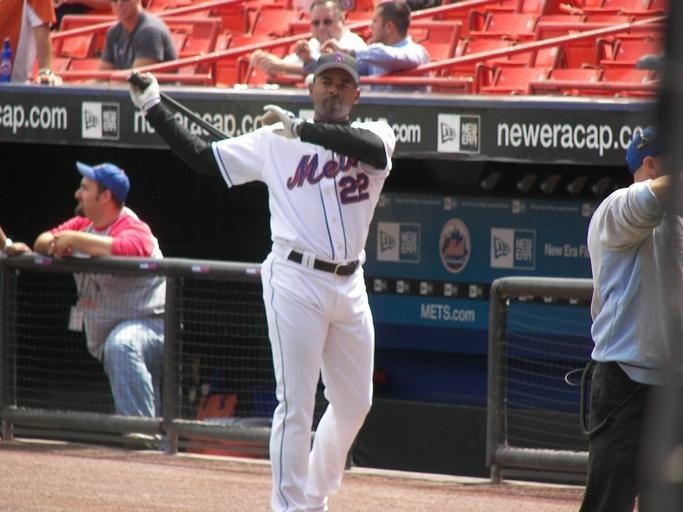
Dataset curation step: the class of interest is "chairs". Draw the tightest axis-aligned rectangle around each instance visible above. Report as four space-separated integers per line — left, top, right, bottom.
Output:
26 1 666 105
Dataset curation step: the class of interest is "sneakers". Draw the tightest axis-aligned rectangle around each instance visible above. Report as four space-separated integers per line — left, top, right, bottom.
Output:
123 432 164 449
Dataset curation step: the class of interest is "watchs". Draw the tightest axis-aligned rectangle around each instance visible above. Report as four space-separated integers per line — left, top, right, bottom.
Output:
38 67 55 75
1 238 12 255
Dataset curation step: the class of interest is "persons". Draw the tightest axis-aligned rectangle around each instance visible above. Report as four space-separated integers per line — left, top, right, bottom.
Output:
0 226 33 258
293 0 432 93
250 0 368 78
0 0 63 85
129 50 396 512
578 127 683 511
90 0 179 86
33 161 167 449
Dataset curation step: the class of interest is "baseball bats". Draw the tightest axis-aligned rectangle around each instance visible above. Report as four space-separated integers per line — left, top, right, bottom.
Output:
127 72 232 139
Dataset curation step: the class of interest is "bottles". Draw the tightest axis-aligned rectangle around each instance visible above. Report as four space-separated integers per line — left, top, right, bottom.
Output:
0 38 12 82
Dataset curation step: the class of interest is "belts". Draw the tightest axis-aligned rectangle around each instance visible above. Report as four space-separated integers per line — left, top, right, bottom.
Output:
288 250 359 275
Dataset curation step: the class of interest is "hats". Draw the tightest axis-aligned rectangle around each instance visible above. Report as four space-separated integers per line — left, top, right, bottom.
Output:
75 161 131 201
312 53 361 85
625 125 668 172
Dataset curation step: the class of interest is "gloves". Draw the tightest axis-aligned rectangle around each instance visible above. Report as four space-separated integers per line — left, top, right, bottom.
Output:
128 72 161 110
261 104 306 138
